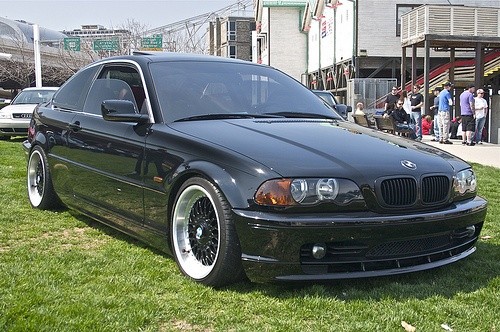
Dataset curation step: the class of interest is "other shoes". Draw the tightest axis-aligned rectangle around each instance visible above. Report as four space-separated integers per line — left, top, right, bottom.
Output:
415 138 421 142
467 142 475 146
431 139 439 141
478 141 483 145
418 135 422 140
439 139 443 143
444 140 453 144
462 142 466 144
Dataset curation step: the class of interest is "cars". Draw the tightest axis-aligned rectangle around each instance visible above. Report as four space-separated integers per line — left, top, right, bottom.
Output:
256 89 353 122
0 86 61 140
21 50 488 290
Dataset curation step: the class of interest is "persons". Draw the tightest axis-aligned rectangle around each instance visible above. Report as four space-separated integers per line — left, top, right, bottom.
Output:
430 81 454 144
384 84 425 142
421 114 487 142
459 84 488 146
354 102 378 130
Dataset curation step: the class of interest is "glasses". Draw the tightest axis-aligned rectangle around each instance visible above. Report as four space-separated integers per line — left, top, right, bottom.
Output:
397 103 403 106
391 89 396 91
479 92 484 94
414 87 418 89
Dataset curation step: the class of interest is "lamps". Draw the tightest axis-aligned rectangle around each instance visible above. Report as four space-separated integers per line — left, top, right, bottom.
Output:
343 64 355 76
331 1 344 9
304 24 311 32
317 14 325 21
327 72 334 80
312 76 320 84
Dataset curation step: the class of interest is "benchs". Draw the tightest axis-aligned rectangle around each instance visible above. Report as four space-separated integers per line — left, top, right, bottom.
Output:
351 113 376 129
372 115 413 137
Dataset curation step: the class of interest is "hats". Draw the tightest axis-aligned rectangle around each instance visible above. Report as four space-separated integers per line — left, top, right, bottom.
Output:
442 82 454 86
431 87 442 91
